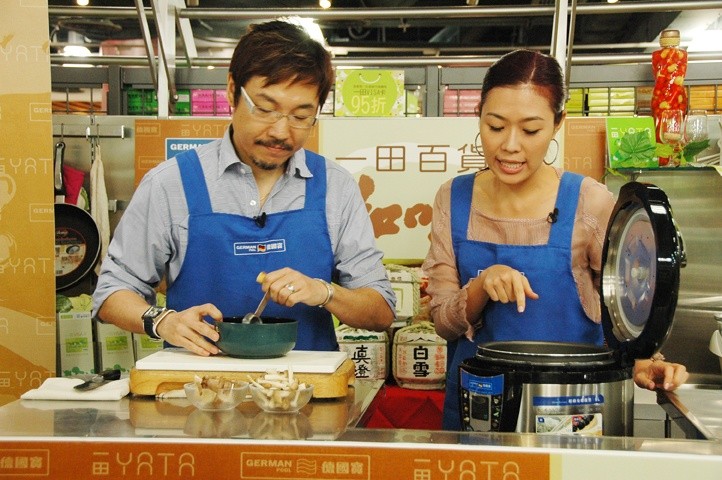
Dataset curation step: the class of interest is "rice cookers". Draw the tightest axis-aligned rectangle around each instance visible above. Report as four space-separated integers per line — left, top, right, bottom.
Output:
458 182 689 449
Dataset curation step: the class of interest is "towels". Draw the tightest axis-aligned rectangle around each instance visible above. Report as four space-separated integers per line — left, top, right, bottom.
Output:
90 157 113 275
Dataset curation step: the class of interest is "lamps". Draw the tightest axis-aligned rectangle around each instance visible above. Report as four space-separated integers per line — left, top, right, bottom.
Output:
62 32 97 68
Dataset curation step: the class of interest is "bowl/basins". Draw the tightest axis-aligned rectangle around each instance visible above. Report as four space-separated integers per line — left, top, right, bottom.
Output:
213 315 300 359
250 383 314 414
183 379 249 411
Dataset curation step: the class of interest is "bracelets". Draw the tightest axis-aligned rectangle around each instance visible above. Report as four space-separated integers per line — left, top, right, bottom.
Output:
312 277 335 307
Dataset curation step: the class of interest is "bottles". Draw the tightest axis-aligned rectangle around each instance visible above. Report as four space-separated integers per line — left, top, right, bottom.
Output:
652 30 688 168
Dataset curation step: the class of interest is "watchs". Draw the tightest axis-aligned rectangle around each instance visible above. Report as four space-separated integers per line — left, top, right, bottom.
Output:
141 304 176 340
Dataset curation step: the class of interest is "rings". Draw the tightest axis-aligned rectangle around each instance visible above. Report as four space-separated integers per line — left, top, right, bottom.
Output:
285 283 296 293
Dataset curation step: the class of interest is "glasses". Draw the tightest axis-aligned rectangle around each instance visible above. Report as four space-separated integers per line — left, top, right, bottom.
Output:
240 87 321 128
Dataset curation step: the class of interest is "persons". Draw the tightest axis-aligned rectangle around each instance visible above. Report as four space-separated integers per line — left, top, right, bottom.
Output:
90 20 396 352
423 48 689 432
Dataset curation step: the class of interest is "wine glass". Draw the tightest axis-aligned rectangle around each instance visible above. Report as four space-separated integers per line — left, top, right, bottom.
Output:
683 108 707 168
659 110 683 168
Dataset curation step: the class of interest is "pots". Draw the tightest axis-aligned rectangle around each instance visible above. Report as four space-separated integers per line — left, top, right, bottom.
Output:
54 142 101 290
